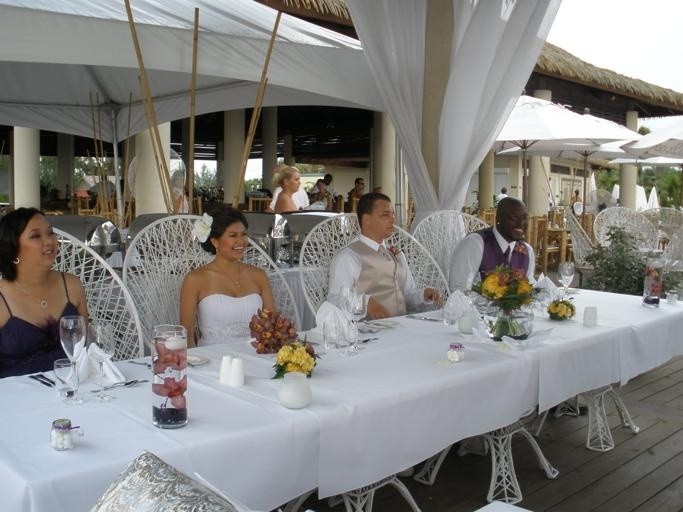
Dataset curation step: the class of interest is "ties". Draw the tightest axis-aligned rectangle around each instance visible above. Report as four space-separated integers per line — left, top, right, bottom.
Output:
378 245 390 262
503 246 510 266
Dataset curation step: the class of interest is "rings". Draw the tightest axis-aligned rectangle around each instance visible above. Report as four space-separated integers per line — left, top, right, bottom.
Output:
440 296 442 298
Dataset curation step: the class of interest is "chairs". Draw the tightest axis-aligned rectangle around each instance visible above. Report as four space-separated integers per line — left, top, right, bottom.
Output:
300 212 450 321
123 213 300 349
566 206 683 276
75 194 95 216
187 194 359 212
0 228 146 357
463 206 566 273
412 209 494 303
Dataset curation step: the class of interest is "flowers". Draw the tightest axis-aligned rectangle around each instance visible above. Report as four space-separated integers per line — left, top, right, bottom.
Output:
481 264 535 307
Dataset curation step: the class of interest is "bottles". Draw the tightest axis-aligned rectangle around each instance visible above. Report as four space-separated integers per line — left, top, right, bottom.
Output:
459 312 479 334
667 289 682 308
279 372 312 409
447 342 465 363
51 419 74 452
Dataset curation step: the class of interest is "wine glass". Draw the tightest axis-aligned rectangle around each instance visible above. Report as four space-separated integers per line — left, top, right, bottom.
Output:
558 261 575 299
54 317 112 405
344 292 367 356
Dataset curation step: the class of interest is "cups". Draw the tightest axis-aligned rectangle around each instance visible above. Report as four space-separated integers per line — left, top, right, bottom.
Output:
323 322 344 352
583 306 598 328
219 355 245 385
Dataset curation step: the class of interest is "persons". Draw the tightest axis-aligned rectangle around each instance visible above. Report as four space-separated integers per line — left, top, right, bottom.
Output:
449 197 536 295
571 190 581 213
327 193 444 478
171 170 189 213
87 176 115 212
0 207 88 378
266 165 365 214
179 206 274 348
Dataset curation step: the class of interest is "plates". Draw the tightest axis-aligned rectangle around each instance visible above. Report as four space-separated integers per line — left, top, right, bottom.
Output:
368 319 400 330
188 352 208 366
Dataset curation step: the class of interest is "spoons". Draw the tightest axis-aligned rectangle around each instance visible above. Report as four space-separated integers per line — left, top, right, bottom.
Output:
127 359 153 370
93 379 138 395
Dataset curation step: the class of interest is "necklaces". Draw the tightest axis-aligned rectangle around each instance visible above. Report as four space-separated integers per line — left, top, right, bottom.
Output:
213 260 240 288
14 274 50 309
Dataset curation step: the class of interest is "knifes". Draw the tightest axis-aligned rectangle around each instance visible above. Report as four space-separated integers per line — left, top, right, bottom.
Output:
406 313 444 324
28 374 52 388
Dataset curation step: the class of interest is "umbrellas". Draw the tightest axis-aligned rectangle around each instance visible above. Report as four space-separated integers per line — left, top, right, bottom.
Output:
490 94 683 226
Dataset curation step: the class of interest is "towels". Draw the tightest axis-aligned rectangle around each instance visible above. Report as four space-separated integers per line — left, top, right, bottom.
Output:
536 273 559 291
73 341 127 385
